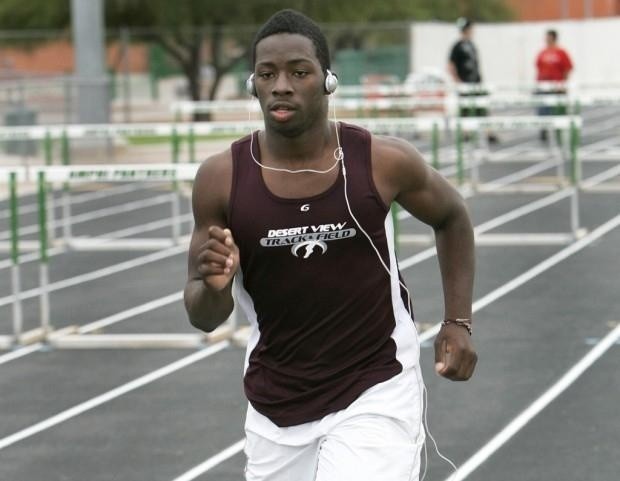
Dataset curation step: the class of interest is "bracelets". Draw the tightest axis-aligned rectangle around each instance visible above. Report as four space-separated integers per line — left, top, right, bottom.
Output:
441 318 472 336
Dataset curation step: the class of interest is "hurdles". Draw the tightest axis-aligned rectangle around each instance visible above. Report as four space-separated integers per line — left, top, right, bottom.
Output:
169 79 591 247
0 122 266 349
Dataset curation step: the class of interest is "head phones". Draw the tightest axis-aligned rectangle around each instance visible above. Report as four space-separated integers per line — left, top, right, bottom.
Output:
245 68 339 97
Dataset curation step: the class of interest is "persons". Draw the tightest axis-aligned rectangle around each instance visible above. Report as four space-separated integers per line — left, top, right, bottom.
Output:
449 17 499 143
184 8 478 481
534 30 573 141
